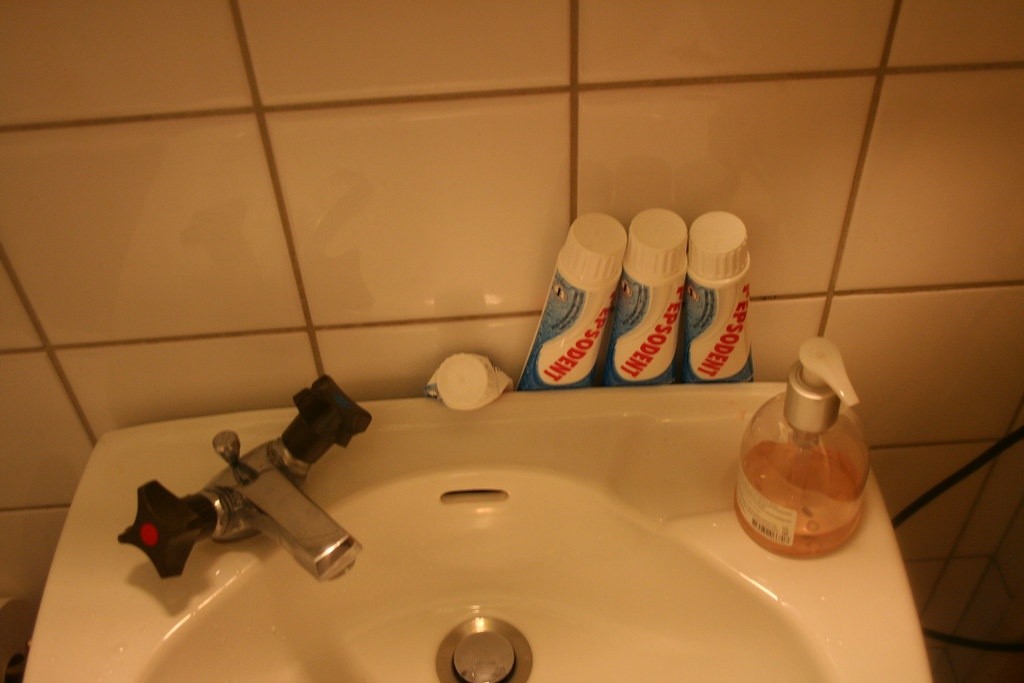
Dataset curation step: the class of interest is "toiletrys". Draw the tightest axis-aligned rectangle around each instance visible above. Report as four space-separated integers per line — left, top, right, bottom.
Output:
732 334 871 560
515 211 629 390
682 211 759 382
421 352 509 410
604 207 690 388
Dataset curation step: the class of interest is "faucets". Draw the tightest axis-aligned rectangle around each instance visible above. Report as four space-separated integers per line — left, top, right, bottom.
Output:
115 372 374 583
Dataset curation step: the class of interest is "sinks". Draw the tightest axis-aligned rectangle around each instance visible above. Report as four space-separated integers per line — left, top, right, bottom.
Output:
19 378 939 683
